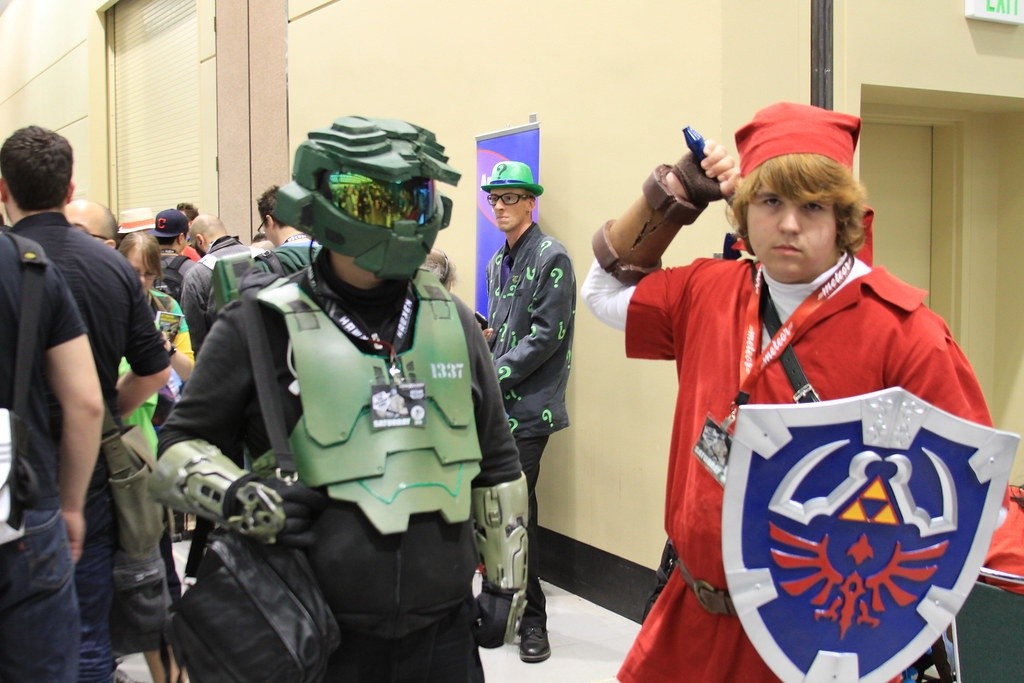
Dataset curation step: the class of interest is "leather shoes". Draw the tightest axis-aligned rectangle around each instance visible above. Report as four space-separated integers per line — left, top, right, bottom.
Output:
519 628 550 663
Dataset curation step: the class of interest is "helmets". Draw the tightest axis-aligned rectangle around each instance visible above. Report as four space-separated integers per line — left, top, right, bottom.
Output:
273 116 461 280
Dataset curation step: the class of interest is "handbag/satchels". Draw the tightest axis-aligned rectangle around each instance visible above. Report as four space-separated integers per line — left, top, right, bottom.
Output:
641 539 679 626
102 423 165 561
168 527 341 683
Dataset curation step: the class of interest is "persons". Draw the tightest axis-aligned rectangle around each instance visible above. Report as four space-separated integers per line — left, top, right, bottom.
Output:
63 200 196 683
0 232 105 683
581 102 1010 683
256 186 323 276
146 110 529 683
180 214 269 467
0 126 170 683
480 160 576 661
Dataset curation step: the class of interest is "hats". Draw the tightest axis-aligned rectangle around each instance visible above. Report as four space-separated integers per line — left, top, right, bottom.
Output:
116 208 155 233
145 209 188 237
481 161 544 197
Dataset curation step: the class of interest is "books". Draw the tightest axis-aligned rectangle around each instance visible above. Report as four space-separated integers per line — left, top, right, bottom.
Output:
155 312 184 345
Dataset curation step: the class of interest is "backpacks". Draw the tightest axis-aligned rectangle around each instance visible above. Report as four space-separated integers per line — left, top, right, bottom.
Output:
0 233 49 544
198 245 265 329
151 255 191 301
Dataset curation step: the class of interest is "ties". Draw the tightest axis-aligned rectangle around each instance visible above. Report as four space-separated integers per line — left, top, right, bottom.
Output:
501 254 511 294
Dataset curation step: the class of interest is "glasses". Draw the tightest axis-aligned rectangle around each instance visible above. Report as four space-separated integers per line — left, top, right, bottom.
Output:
258 214 274 238
487 193 534 206
71 223 108 241
134 268 160 280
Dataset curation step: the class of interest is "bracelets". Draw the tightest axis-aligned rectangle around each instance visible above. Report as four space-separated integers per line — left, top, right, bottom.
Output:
169 343 177 358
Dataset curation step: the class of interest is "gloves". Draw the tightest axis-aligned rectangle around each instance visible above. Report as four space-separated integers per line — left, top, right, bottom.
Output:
221 471 329 548
471 580 518 648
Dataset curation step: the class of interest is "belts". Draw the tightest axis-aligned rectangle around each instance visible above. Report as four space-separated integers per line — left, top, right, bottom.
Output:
675 559 738 617
